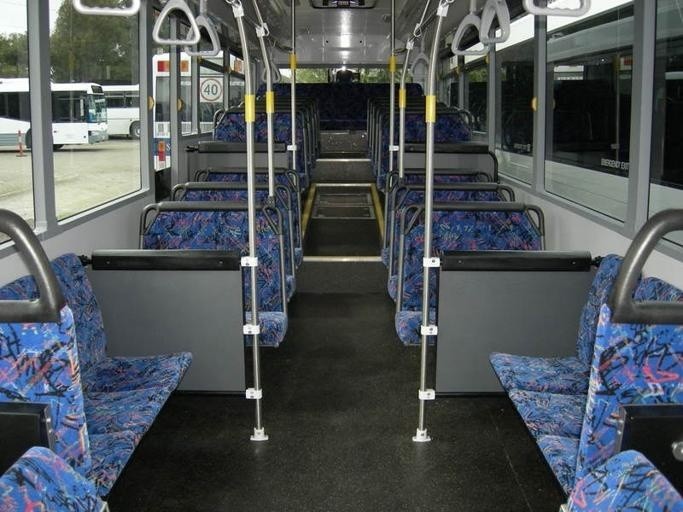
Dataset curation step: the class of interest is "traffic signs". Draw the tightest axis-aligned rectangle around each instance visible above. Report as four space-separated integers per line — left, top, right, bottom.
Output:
200 80 221 100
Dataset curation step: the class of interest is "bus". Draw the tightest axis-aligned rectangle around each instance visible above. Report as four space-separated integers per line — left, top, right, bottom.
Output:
0 79 140 149
151 51 251 171
